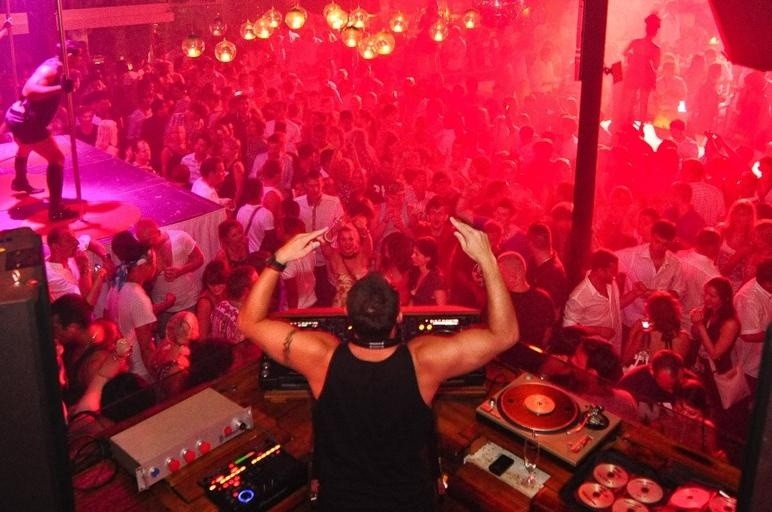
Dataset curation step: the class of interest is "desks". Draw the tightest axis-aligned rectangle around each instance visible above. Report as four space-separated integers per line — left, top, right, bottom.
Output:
73 357 744 512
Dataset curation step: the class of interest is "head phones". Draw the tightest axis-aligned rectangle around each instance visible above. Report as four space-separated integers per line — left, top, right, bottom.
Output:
335 316 404 350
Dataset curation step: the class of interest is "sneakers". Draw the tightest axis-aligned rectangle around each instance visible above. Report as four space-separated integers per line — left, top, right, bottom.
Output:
49 209 79 220
10 179 44 193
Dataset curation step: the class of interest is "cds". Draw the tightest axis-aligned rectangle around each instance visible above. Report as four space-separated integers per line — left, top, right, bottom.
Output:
576 463 738 512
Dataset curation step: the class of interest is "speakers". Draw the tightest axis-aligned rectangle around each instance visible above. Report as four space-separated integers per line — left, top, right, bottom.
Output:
0 228 75 512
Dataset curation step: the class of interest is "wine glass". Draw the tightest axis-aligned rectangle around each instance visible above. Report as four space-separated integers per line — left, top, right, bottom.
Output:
522 440 541 488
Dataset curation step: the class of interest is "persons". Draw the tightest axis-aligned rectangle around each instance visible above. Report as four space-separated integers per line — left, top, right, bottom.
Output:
3 2 771 512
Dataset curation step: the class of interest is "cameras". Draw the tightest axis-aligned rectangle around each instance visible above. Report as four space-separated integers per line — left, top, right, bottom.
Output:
642 321 650 328
